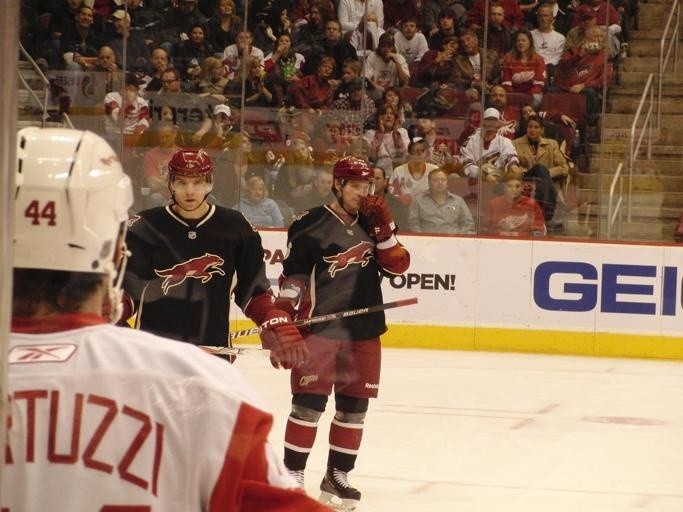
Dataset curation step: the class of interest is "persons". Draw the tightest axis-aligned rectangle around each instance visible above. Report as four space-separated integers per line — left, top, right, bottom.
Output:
118 147 313 370
274 153 411 502
3 125 332 512
17 0 636 239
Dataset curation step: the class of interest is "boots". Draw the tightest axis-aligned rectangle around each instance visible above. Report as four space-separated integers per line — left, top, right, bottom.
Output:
286 466 361 501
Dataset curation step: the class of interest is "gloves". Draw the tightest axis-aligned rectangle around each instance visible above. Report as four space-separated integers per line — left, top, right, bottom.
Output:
102 289 135 325
244 279 310 369
359 196 399 242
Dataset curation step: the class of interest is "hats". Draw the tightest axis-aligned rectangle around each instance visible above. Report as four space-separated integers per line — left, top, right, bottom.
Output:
113 5 597 153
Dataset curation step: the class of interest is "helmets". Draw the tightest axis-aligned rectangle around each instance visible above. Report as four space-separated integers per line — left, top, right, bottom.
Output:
11 126 134 279
167 147 214 195
332 156 376 198
